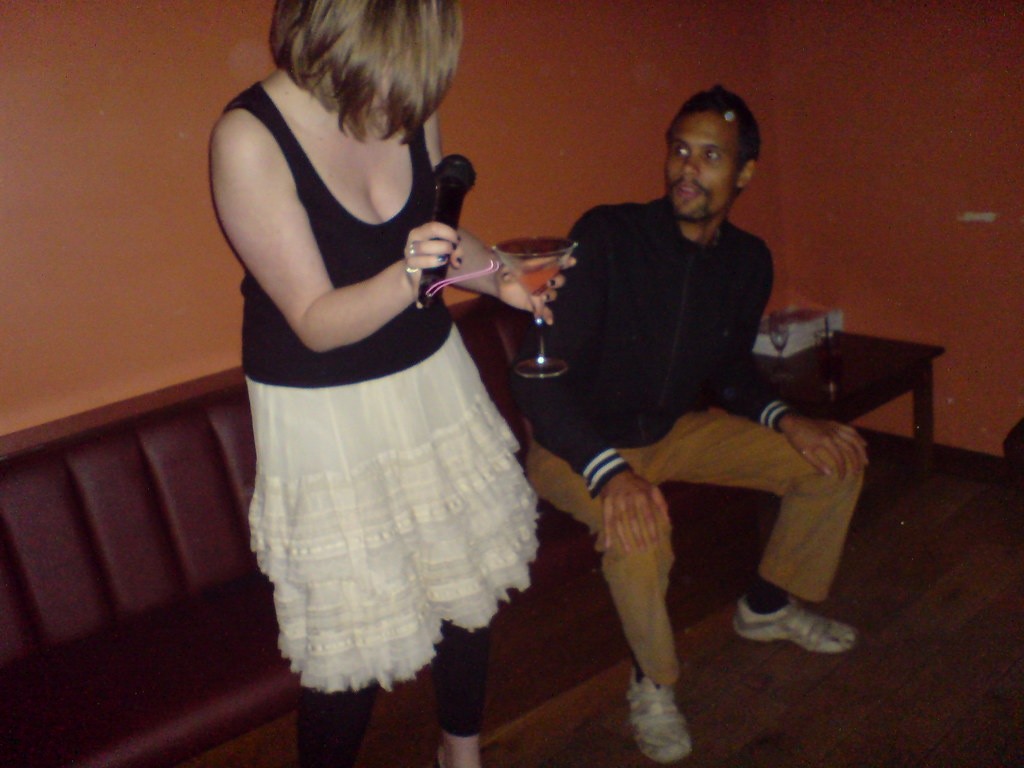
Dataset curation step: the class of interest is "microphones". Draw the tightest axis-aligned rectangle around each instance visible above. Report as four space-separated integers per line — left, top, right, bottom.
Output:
416 154 476 310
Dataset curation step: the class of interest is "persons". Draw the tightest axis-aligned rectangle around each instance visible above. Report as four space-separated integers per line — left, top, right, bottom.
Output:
525 84 869 764
209 0 576 768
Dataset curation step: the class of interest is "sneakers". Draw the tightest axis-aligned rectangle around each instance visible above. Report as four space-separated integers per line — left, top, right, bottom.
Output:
732 592 859 653
624 666 693 764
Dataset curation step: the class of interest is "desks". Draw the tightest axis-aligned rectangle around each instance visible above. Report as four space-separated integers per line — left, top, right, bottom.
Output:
754 330 945 476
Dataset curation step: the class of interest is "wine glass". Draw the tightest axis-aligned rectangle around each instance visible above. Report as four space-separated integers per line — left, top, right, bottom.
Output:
492 237 578 380
767 316 790 373
814 330 839 393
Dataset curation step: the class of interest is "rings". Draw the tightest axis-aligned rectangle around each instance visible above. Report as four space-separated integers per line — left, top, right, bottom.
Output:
409 244 416 255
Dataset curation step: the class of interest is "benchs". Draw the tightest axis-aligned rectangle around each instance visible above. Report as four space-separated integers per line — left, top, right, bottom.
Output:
0 289 780 768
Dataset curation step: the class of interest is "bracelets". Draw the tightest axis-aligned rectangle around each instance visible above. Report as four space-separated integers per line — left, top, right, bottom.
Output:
400 258 420 272
